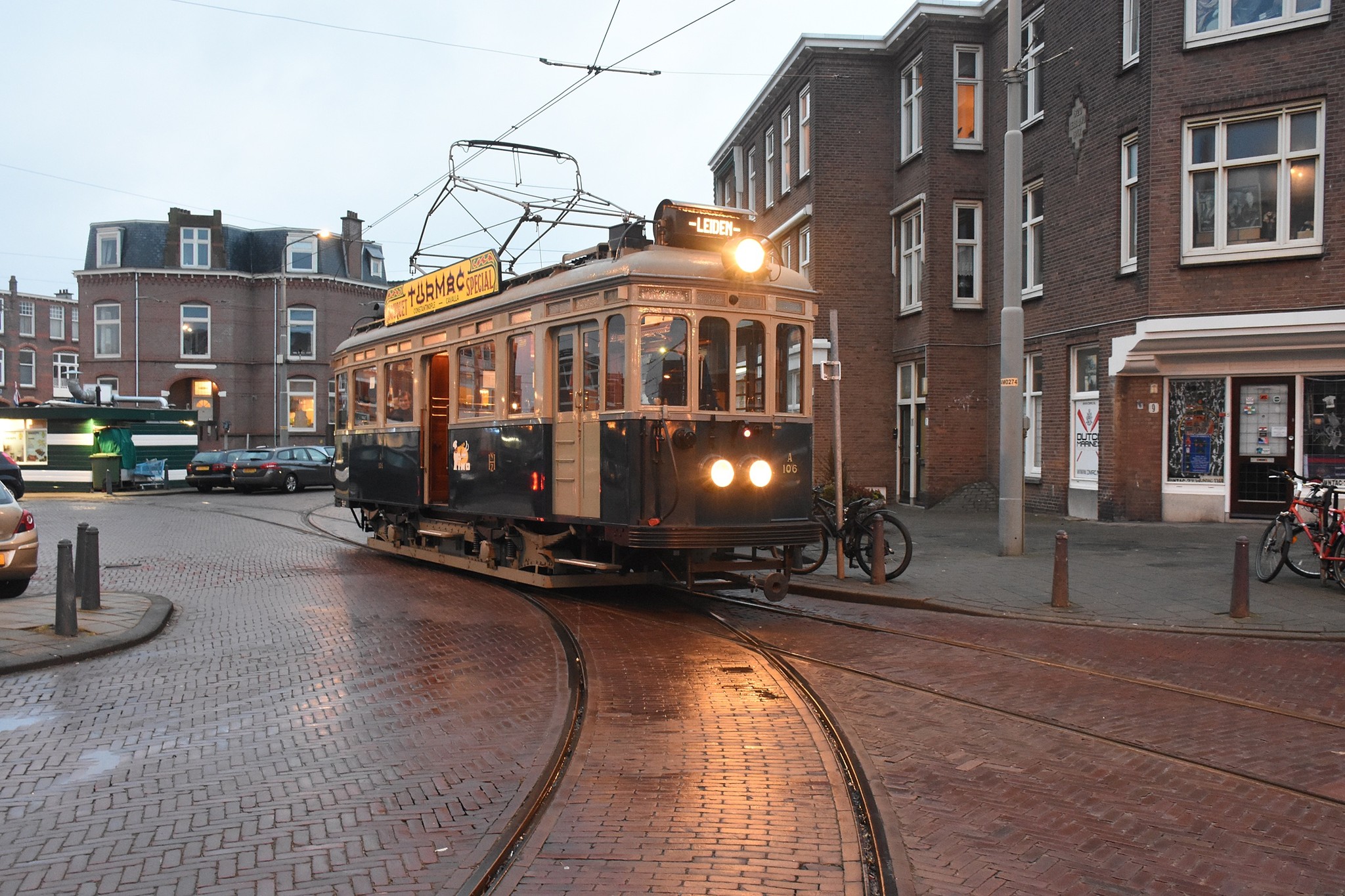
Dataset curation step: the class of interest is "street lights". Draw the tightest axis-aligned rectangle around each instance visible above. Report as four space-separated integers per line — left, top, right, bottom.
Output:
279 228 330 447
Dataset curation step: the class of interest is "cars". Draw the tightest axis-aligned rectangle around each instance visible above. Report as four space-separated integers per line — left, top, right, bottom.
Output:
184 449 246 492
0 478 40 600
0 450 25 505
301 444 335 460
228 445 335 493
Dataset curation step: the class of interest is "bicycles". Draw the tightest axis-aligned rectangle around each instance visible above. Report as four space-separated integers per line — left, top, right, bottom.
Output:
770 475 913 579
1253 468 1345 592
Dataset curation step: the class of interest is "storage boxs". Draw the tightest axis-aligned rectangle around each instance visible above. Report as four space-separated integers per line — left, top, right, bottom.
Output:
1297 231 1314 239
1195 227 1271 248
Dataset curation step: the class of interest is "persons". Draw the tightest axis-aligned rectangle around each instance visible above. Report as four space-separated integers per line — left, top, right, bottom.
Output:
645 318 715 411
1227 190 1258 228
387 391 413 422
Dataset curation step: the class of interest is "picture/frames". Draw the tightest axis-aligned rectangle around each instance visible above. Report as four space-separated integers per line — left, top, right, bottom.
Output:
1196 183 1263 233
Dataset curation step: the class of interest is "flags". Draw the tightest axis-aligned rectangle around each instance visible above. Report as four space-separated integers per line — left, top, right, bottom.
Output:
13 391 21 406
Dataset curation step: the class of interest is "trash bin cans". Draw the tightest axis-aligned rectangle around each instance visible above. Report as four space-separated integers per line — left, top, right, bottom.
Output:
89 453 122 490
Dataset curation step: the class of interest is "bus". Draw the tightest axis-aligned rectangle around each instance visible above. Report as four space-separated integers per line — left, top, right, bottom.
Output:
333 137 824 605
